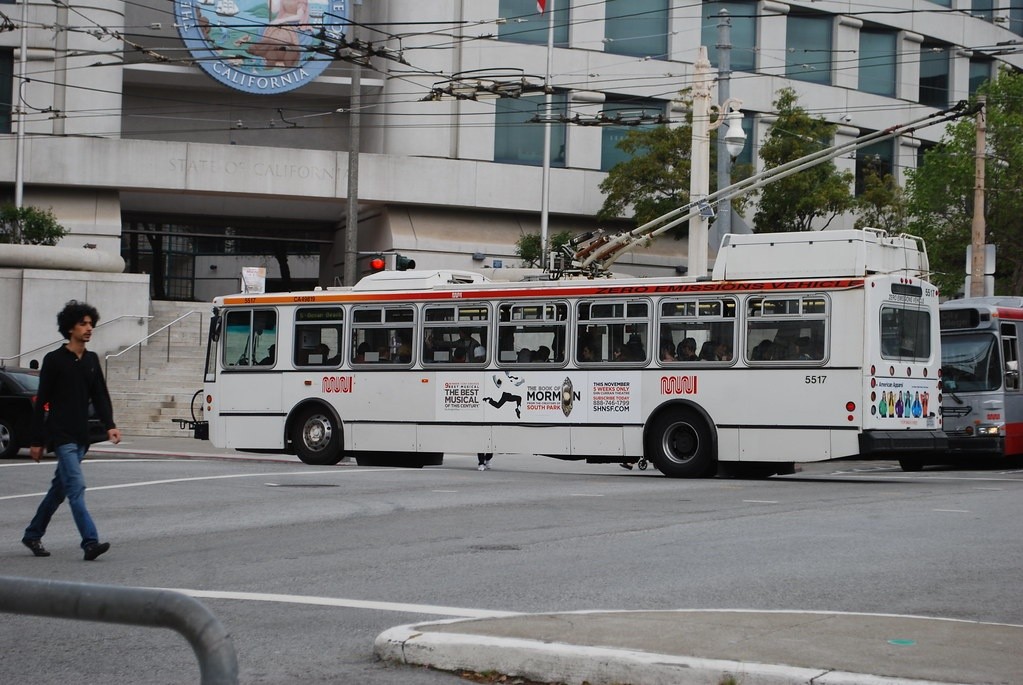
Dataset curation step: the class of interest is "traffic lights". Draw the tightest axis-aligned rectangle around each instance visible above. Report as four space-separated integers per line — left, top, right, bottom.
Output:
396 256 416 271
370 254 386 274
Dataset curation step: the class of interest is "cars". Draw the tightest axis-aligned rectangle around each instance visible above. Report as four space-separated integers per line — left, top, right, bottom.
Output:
0 367 47 460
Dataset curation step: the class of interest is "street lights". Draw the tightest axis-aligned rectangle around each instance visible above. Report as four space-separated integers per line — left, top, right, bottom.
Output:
688 45 747 278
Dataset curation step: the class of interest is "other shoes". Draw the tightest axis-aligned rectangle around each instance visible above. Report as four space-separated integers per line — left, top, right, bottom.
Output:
20 536 51 556
84 541 111 560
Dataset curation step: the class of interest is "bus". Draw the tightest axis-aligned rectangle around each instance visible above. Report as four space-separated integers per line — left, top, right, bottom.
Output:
202 227 949 478
899 296 1023 470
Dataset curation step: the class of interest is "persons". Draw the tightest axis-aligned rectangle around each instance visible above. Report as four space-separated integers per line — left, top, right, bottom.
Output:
794 336 819 360
475 452 493 471
21 299 121 561
428 326 485 362
353 329 412 364
752 339 775 361
257 344 275 365
30 359 39 369
661 337 732 361
880 391 928 418
516 345 551 362
579 333 646 362
